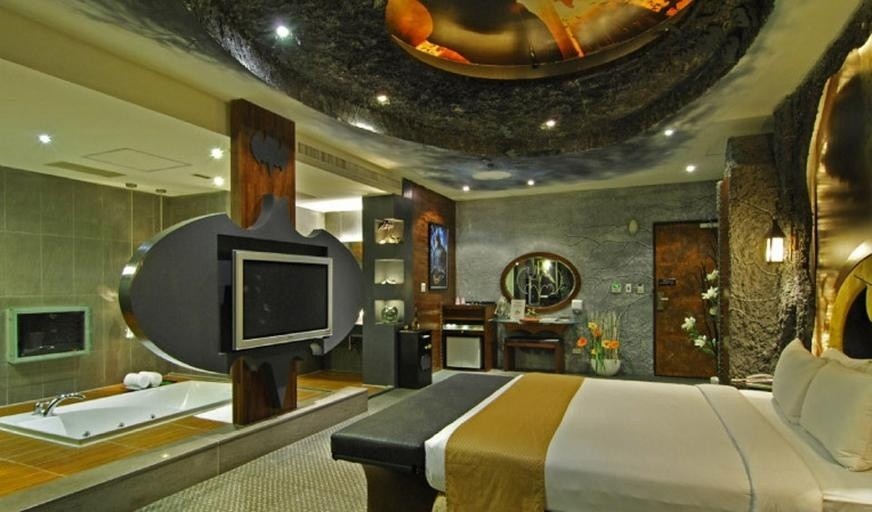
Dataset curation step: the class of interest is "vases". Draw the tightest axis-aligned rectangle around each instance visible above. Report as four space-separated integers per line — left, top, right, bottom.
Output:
586 359 622 376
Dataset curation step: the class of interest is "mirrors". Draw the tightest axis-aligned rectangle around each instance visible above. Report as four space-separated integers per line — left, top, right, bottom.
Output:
500 252 581 313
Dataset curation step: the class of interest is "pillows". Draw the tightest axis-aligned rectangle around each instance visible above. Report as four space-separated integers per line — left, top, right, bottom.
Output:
770 336 872 473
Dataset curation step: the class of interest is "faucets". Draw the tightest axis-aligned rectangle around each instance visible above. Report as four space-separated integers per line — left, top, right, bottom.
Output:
43 391 87 416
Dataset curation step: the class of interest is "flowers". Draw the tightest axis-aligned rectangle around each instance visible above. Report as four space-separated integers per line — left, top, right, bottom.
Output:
574 310 623 377
680 269 719 375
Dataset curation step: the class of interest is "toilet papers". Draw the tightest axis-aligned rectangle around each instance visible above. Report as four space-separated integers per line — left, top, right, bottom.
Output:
571 299 584 314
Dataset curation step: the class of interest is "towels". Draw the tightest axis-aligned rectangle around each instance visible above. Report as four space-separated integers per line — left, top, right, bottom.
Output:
124 370 163 390
124 370 164 390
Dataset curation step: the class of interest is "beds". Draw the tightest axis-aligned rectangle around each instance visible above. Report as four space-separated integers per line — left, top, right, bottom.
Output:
329 372 872 512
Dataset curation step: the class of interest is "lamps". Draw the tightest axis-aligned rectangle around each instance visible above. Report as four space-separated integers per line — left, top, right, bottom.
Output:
765 218 786 264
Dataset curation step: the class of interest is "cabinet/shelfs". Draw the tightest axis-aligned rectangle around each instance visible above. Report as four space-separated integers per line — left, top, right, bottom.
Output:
441 301 497 371
362 195 434 390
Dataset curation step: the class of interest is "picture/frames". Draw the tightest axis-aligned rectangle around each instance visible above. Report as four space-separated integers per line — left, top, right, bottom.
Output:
428 220 450 291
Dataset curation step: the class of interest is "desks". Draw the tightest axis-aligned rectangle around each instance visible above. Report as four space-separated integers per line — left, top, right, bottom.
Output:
489 317 579 338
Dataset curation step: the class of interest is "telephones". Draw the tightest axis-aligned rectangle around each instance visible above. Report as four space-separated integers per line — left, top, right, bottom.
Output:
745 373 773 385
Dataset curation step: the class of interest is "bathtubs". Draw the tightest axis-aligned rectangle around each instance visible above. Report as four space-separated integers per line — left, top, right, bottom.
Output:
0 378 233 445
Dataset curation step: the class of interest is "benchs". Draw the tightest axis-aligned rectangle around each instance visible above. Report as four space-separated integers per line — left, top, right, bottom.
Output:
503 336 564 373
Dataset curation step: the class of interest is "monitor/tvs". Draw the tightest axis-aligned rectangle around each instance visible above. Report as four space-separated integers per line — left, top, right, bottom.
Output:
230 248 334 352
